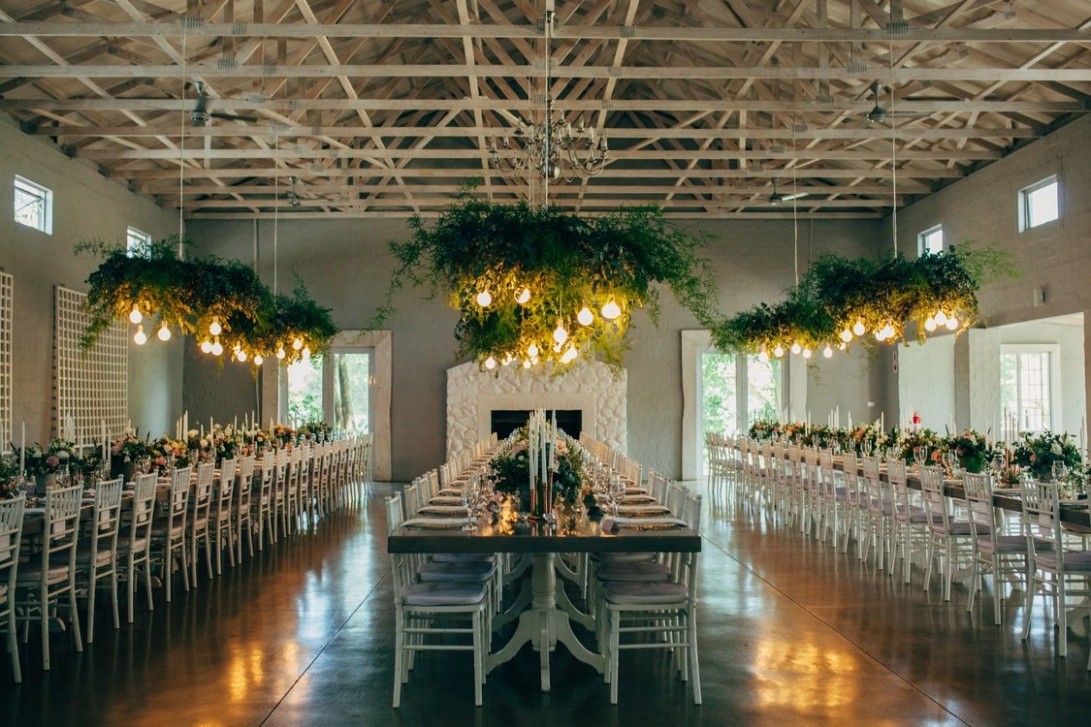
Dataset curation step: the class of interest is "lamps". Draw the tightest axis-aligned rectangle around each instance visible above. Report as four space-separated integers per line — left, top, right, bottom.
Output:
71 0 1022 382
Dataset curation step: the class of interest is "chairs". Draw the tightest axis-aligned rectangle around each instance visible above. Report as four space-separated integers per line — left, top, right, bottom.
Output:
706 431 1090 669
0 432 373 680
381 430 704 706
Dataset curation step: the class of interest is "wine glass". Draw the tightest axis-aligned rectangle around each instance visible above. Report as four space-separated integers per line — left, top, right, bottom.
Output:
462 425 627 531
733 425 1089 509
4 425 357 524
